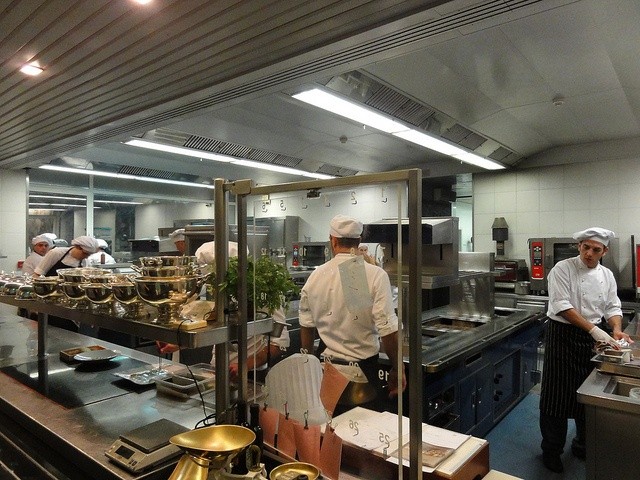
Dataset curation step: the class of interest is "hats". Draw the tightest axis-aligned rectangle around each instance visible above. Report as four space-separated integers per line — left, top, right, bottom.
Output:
358 242 369 248
329 215 363 238
41 233 58 240
194 240 250 262
71 236 99 253
31 234 53 246
54 239 68 247
96 239 108 250
572 228 616 247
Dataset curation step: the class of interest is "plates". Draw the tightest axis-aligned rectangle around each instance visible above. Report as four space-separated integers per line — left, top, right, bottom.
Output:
71 349 122 364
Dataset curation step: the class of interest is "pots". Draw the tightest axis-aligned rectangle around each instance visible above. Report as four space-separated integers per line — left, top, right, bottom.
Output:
34 254 213 325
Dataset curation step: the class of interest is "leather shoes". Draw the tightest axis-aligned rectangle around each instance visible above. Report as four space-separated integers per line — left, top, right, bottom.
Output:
542 452 564 473
571 445 586 460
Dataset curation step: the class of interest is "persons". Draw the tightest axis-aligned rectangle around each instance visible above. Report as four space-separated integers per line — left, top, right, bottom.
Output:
169 228 185 256
540 227 630 474
85 238 116 267
33 236 96 278
156 240 290 384
299 221 406 418
22 235 53 276
358 246 376 265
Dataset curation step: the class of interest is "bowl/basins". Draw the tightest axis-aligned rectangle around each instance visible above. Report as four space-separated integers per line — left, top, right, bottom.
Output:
1 275 34 303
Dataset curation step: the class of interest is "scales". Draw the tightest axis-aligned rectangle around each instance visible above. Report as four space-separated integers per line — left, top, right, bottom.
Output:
168 423 256 480
104 418 197 474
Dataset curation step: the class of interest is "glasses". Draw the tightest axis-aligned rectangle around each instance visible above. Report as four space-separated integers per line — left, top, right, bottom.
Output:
37 244 48 247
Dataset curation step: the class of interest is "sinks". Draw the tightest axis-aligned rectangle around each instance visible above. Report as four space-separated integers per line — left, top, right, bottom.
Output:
421 315 489 332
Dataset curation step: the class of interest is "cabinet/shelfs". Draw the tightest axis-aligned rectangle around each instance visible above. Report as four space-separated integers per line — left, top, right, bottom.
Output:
426 386 459 428
493 351 521 414
457 365 492 432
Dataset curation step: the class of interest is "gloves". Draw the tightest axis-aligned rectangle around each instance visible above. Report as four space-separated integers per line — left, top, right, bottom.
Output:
589 326 620 351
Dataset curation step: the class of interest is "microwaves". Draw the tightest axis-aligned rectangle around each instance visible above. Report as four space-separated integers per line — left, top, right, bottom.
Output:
526 236 609 303
